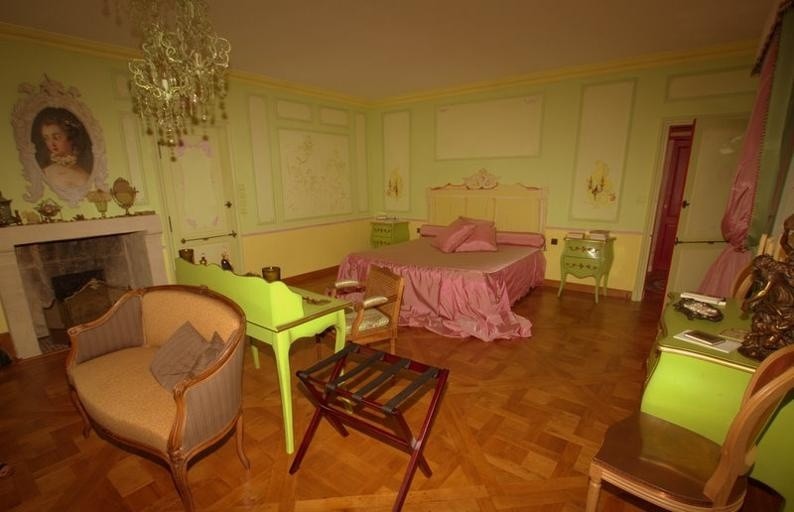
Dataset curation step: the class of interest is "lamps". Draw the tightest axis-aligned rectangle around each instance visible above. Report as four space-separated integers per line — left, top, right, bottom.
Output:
124 0 233 162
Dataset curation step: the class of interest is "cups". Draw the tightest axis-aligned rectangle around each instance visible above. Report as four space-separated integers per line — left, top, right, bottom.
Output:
178 249 194 264
261 267 281 281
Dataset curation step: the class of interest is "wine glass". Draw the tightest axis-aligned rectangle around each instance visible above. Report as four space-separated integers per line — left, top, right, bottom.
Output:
93 200 107 218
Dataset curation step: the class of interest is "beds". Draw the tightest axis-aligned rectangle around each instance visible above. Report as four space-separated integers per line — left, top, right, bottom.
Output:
322 168 551 343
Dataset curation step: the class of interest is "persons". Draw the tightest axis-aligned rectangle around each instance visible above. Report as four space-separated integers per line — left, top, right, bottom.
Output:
37 113 89 192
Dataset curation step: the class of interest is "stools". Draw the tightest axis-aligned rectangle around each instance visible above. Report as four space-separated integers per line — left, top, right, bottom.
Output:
287 342 450 512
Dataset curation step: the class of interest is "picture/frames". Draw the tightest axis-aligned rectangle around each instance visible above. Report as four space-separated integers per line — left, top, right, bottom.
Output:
14 70 108 211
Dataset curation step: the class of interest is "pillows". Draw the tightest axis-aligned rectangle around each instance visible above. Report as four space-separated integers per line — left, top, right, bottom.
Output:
182 328 225 379
152 317 207 393
454 220 498 252
429 216 479 254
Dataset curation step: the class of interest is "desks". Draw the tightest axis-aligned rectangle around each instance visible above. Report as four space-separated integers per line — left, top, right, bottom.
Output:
173 255 354 456
636 290 794 511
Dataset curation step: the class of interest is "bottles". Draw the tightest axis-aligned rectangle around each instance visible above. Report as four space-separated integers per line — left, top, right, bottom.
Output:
199 253 206 265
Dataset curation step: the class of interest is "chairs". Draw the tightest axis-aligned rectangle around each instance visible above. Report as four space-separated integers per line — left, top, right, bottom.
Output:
582 343 794 511
64 282 251 512
316 263 405 355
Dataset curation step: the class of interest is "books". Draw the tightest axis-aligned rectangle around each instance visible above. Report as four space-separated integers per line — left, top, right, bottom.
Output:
374 214 388 221
673 326 751 353
683 329 727 346
681 291 727 307
566 230 585 239
588 230 610 241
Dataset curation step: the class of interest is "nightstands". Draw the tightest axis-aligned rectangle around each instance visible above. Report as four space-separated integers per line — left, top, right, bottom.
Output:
556 237 617 305
367 217 411 248
